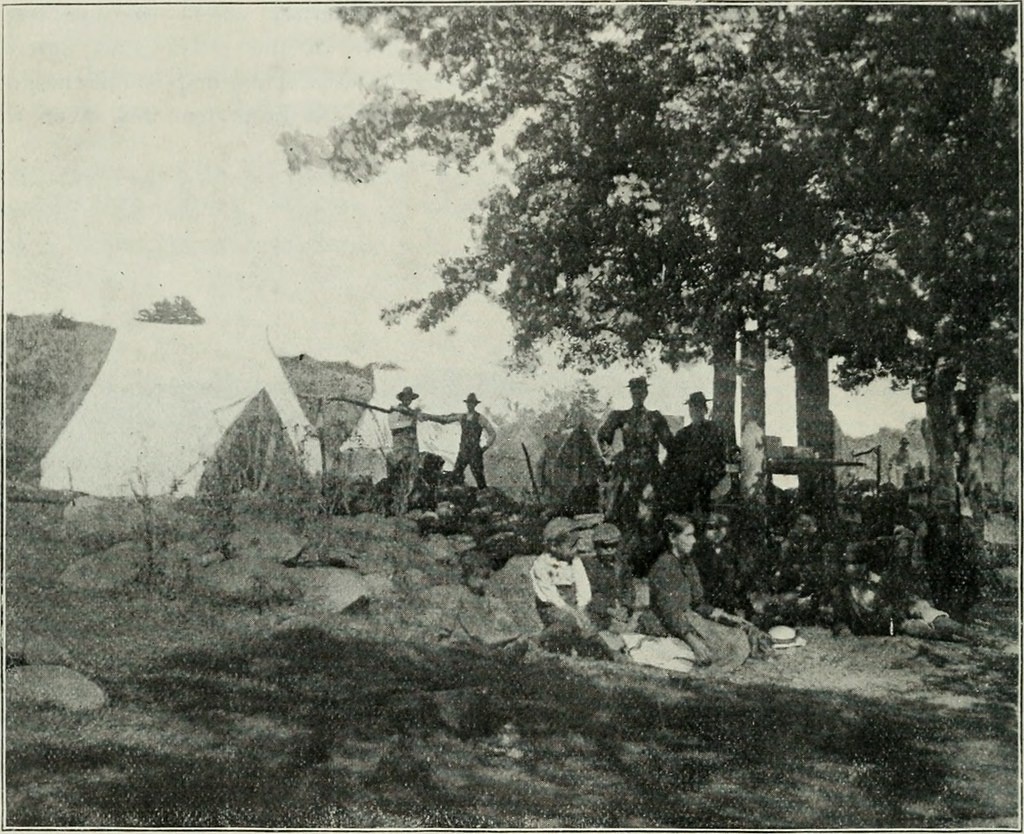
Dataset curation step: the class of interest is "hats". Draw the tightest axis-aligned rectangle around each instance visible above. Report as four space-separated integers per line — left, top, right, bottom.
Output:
931 487 957 505
626 376 651 387
464 392 481 403
397 387 419 400
542 517 582 545
683 392 714 404
840 547 873 563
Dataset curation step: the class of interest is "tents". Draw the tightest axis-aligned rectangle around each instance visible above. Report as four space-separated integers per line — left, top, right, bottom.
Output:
41 315 324 501
339 368 498 470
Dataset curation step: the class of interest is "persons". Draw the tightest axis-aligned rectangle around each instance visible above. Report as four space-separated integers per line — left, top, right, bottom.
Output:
656 391 727 524
913 483 986 624
647 515 747 664
888 438 912 486
598 377 674 523
418 393 496 490
831 551 1007 650
531 516 594 628
388 386 420 453
698 458 936 631
585 523 636 624
